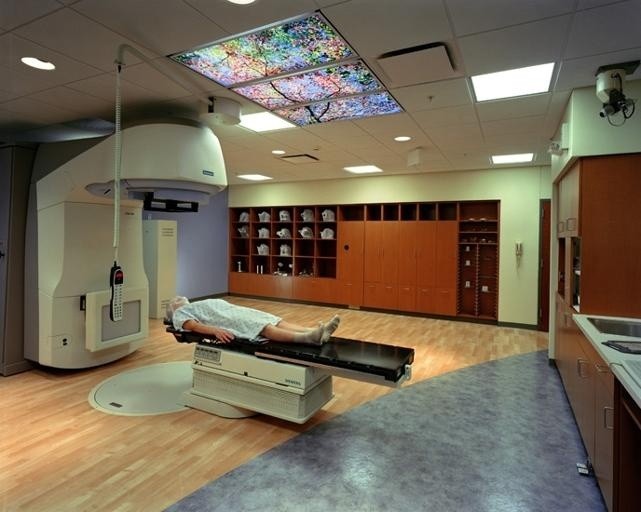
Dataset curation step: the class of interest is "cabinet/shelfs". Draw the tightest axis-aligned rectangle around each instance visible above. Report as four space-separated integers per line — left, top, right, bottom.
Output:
229 199 501 326
554 155 641 511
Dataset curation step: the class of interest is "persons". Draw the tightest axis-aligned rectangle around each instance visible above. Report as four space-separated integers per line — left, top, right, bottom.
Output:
166 296 340 346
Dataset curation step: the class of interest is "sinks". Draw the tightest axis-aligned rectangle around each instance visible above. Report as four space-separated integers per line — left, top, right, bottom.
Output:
587 317 641 337
